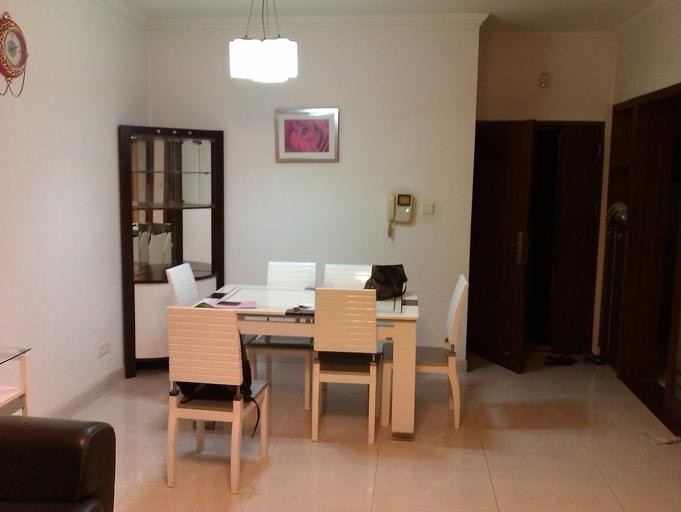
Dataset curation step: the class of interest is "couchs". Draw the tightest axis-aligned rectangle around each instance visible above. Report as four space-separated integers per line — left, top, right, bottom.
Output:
1 412 121 511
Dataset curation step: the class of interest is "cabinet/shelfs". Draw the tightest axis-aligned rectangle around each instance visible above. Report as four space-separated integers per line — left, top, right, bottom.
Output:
664 281 681 422
119 123 228 381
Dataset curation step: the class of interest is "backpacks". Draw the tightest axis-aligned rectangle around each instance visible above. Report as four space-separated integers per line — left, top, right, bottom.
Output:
176 303 252 403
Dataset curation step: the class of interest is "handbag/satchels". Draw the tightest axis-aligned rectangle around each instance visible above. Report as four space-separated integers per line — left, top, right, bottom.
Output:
364 264 407 301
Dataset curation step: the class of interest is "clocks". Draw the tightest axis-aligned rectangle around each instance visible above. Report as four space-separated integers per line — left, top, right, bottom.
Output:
1 11 31 98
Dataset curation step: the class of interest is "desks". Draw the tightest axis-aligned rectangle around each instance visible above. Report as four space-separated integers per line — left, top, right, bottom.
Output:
1 341 30 419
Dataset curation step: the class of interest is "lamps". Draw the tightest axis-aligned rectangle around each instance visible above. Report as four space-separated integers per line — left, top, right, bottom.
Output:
224 0 302 84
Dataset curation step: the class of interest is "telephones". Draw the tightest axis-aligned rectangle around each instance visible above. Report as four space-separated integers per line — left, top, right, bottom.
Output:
387 193 412 223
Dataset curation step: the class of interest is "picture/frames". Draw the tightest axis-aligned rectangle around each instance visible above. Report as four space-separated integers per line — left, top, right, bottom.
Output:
273 106 342 165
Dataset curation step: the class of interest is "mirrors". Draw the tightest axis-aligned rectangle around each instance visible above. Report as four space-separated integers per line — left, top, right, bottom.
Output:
130 135 174 277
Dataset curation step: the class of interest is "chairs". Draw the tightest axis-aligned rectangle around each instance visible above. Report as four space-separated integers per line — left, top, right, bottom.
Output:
164 302 272 493
164 260 206 308
312 285 377 446
382 270 473 430
245 257 319 412
304 261 397 415
